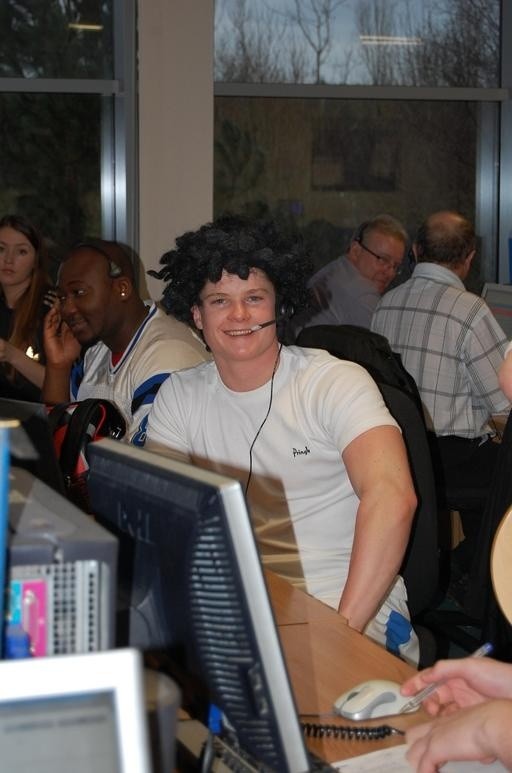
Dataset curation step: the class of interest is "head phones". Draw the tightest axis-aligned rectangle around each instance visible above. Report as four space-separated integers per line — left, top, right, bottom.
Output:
242 252 311 330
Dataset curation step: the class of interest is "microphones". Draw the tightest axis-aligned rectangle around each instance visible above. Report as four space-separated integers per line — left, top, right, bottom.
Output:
249 314 285 334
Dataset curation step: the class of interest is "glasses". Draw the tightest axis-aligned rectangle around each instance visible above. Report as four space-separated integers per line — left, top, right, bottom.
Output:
358 242 404 276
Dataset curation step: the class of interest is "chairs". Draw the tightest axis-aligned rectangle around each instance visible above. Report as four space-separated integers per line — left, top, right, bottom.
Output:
295 323 484 654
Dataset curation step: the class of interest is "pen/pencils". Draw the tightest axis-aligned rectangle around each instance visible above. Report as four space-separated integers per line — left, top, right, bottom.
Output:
399 642 493 715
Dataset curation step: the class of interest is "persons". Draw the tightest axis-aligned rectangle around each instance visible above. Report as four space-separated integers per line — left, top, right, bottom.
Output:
398 654 512 772
294 211 410 332
1 214 60 406
368 211 511 650
142 213 421 668
39 237 211 447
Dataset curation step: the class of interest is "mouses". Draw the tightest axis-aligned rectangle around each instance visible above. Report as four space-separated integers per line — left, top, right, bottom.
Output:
331 677 423 722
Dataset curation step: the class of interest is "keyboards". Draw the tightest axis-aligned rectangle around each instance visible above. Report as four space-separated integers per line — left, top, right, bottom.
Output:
180 723 344 773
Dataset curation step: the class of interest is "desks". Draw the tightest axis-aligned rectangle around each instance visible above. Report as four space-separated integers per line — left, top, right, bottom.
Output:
261 564 443 773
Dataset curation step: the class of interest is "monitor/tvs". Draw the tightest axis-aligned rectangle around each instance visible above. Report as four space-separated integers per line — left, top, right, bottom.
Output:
0 646 155 773
85 432 320 769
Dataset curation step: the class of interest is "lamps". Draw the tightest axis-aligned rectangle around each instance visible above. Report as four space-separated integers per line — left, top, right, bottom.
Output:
67 0 105 33
